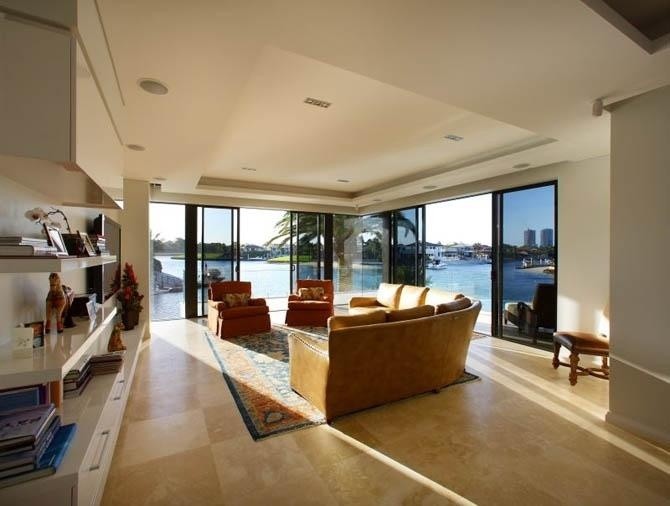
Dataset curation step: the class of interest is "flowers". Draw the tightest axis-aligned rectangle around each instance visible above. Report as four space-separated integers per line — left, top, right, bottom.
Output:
24 204 72 232
117 283 135 316
122 261 145 315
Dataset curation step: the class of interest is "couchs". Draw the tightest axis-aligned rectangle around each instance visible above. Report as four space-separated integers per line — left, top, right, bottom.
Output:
207 281 270 339
286 282 482 427
284 280 334 328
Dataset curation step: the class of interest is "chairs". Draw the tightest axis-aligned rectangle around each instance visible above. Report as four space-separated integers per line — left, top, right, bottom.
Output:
504 283 556 344
552 297 610 387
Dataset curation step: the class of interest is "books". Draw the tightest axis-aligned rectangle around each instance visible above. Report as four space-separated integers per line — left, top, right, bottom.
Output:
62 351 93 399
62 233 110 256
0 403 77 489
0 236 48 245
0 245 58 256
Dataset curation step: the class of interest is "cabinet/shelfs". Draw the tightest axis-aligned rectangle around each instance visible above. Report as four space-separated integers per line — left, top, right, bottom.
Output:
1 254 127 506
1 0 127 210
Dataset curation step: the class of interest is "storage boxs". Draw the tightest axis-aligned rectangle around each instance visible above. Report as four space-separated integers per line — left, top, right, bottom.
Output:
69 292 97 318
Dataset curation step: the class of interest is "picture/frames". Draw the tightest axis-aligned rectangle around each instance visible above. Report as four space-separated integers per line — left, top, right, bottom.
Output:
76 230 98 257
44 223 70 256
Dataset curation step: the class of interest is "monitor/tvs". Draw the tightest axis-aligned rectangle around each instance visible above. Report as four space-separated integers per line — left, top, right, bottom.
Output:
90 214 121 304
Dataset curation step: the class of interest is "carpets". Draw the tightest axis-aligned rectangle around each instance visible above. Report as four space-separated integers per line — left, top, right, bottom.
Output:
201 304 481 442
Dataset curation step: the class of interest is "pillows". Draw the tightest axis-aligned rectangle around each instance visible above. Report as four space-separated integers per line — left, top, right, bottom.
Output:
223 293 251 308
298 286 325 301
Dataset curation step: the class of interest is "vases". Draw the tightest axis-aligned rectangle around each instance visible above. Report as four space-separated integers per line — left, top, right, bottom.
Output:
129 310 139 326
120 312 135 331
